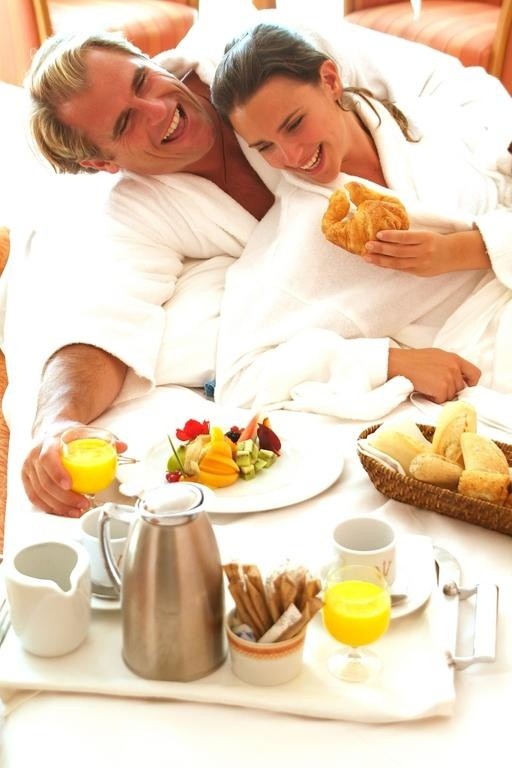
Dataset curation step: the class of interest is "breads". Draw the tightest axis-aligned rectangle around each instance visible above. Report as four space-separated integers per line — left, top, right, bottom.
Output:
320 180 409 256
433 406 477 463
408 453 461 485
458 467 509 505
366 415 435 477
457 432 509 475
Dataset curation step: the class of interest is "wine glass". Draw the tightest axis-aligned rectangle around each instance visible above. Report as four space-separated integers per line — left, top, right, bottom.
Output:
322 563 393 682
59 425 118 516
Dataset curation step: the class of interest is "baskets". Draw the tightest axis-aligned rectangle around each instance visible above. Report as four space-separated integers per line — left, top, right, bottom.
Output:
357 422 512 537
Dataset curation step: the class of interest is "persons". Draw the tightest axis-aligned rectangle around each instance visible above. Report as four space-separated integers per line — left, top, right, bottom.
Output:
20 11 512 519
210 21 511 440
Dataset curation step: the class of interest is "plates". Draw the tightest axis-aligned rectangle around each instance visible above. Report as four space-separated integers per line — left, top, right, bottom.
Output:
313 545 441 620
110 408 345 513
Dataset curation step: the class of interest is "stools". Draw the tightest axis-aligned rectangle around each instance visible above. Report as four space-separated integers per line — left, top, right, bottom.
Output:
342 0 512 94
0 0 201 89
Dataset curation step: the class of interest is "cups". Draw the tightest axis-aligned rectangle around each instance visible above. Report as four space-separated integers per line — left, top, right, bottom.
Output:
332 519 397 585
4 534 94 657
224 606 307 687
76 505 132 597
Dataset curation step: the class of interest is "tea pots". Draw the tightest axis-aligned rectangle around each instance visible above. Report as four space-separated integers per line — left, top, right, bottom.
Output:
96 482 232 682
